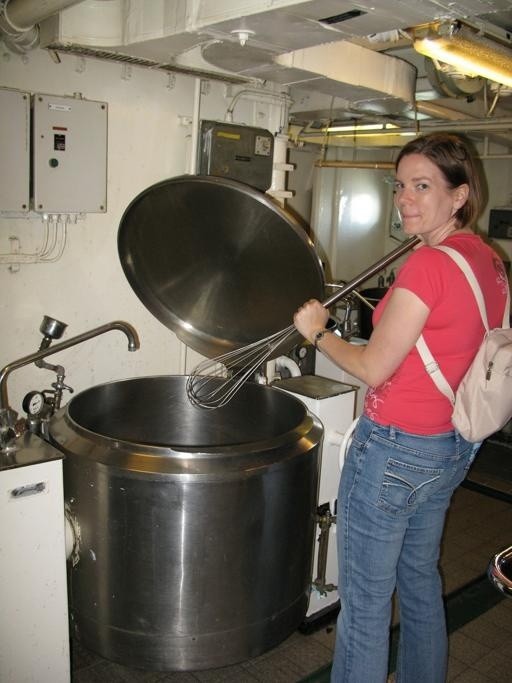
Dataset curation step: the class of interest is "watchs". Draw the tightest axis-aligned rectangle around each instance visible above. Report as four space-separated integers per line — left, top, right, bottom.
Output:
315 328 331 348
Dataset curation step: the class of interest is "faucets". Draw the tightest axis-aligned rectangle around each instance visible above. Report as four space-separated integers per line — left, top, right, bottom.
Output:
0 322 140 410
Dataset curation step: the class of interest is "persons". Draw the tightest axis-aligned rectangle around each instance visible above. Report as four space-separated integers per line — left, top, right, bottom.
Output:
293 131 512 683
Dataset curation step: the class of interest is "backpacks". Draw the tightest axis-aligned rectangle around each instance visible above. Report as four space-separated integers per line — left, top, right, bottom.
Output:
414 244 512 443
